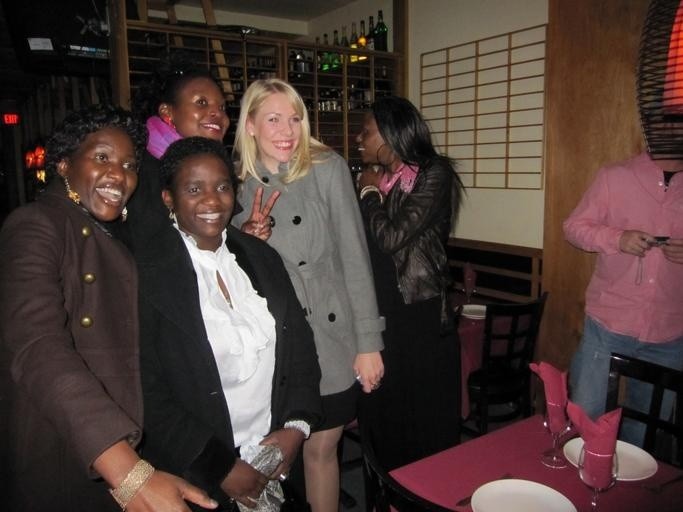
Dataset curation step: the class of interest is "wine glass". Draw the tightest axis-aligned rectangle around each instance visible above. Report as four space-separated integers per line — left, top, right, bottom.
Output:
540 399 572 470
575 443 618 512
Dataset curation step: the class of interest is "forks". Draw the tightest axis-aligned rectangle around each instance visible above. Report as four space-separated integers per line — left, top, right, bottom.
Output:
644 473 683 493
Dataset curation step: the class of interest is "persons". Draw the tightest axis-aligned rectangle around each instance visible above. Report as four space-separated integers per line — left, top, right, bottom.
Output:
0 107 218 510
230 78 385 510
561 103 682 448
353 93 468 443
139 137 326 511
134 71 281 243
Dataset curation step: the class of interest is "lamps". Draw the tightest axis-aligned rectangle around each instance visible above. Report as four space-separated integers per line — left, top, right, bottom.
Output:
633 1 682 194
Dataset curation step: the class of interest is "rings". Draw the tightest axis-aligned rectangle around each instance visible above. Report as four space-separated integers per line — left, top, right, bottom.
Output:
279 470 287 481
370 379 380 390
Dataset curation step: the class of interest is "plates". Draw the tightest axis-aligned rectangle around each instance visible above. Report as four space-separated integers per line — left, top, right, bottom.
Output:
456 303 491 321
562 436 658 482
469 478 578 512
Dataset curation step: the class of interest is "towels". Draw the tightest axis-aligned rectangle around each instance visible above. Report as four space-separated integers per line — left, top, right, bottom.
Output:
564 399 623 486
526 361 568 433
462 262 477 293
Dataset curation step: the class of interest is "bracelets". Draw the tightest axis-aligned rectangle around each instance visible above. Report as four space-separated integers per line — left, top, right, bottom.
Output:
359 184 384 204
286 417 311 438
108 460 155 508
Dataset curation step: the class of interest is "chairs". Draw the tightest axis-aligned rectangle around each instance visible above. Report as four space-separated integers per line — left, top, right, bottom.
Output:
604 353 683 469
358 445 457 511
461 290 548 439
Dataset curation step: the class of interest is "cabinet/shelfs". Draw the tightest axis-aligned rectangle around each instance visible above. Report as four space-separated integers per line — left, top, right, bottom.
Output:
105 0 410 172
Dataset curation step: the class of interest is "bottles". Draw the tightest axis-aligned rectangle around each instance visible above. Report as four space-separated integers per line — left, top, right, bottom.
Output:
308 65 389 113
229 49 276 94
313 9 388 73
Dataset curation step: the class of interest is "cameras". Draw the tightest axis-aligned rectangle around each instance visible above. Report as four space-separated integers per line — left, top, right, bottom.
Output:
643 237 670 247
